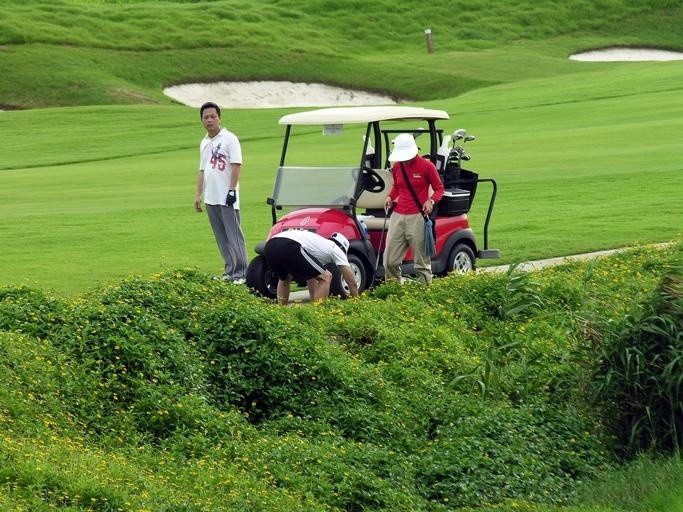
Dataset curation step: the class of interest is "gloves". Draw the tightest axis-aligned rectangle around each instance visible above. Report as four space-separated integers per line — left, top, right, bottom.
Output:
225 189 237 207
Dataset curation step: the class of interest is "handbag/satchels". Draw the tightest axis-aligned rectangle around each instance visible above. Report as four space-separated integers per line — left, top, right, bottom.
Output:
424 220 436 256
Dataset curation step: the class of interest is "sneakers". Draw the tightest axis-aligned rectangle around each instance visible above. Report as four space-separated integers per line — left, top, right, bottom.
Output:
221 273 248 286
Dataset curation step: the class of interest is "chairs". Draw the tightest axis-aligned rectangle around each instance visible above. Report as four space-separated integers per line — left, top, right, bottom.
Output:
349 168 398 231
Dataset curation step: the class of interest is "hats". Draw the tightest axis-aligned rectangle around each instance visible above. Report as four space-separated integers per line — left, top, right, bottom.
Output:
330 232 350 256
388 133 418 162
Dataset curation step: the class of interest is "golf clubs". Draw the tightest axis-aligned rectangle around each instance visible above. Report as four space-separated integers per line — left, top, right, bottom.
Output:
448 129 475 163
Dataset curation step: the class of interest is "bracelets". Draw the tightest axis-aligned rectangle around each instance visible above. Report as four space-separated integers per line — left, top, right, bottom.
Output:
429 198 434 206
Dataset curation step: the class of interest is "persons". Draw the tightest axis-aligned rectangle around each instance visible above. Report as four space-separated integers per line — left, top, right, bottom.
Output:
192 102 248 286
382 134 445 284
262 229 358 306
